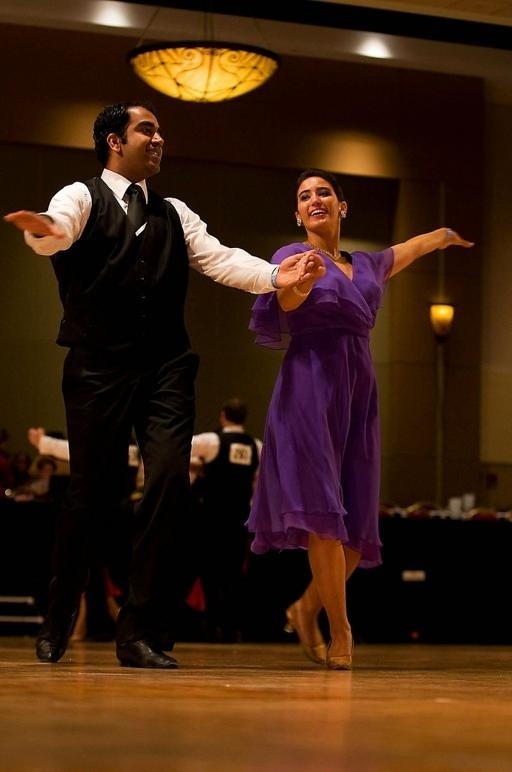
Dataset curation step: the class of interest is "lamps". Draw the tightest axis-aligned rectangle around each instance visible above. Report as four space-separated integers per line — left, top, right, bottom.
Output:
423 293 458 342
122 0 280 108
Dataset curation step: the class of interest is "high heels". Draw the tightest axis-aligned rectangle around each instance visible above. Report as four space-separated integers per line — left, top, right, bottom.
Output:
285 603 328 666
327 639 354 670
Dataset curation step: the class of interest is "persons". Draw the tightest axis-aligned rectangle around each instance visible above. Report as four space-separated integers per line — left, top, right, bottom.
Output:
6 104 312 668
0 448 57 501
245 170 473 670
171 397 262 631
27 427 139 515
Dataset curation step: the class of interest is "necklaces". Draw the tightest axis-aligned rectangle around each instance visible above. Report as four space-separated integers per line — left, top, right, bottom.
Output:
304 240 343 261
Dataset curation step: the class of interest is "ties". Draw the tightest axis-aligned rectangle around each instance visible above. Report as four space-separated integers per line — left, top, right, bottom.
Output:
126 185 146 235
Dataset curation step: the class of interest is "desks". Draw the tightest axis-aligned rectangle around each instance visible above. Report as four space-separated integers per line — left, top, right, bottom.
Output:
369 509 509 646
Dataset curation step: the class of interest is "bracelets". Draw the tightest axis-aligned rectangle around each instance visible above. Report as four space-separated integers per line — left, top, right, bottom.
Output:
272 263 279 290
446 223 452 243
292 285 313 298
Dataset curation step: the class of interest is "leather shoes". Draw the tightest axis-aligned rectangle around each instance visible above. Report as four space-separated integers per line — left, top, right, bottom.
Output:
116 637 179 668
36 605 81 663
